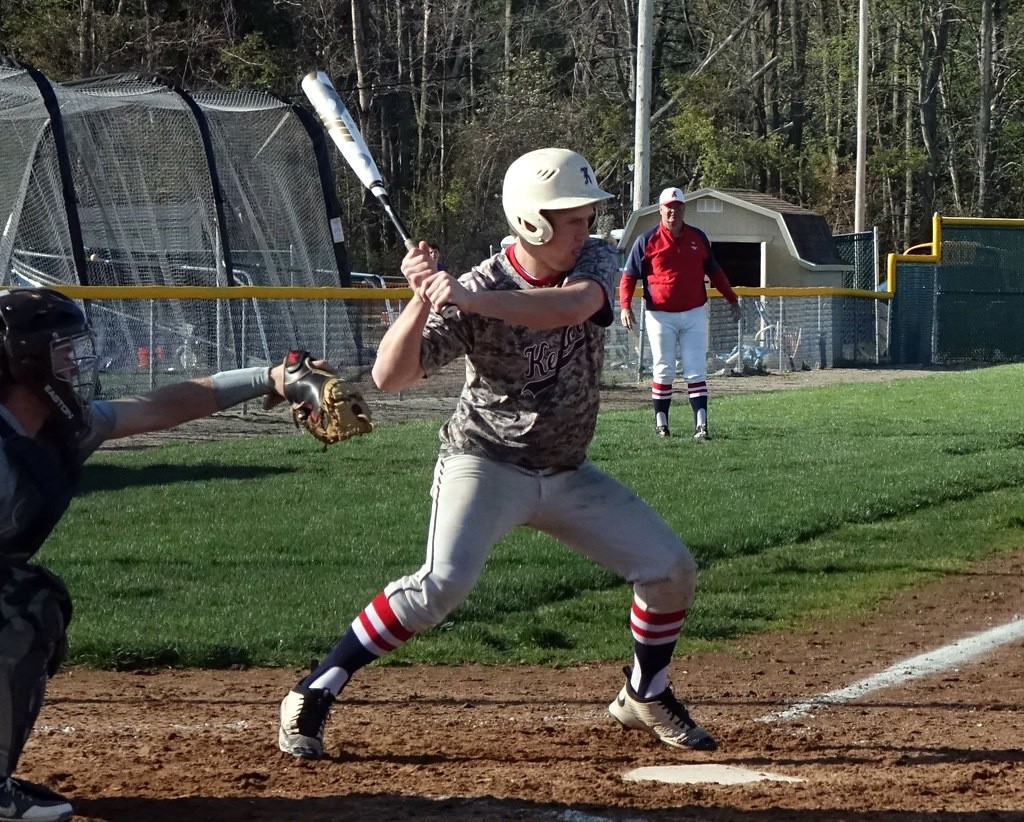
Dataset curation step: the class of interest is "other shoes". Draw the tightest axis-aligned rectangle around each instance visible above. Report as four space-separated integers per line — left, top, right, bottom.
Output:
656 426 669 437
695 428 710 441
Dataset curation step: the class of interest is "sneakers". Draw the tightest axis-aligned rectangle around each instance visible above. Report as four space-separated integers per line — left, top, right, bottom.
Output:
278 675 336 759
609 667 718 751
0 778 74 822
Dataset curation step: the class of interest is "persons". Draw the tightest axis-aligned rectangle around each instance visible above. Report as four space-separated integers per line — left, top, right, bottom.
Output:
429 245 444 271
133 318 170 372
278 148 717 758
0 287 375 822
620 187 744 439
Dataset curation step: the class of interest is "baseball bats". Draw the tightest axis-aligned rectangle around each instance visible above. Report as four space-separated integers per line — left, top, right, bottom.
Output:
300 70 458 319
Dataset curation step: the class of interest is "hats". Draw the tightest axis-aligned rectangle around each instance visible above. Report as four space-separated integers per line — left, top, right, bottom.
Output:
659 187 685 206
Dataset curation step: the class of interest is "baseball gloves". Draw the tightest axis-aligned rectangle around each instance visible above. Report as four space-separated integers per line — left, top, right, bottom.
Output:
281 347 374 445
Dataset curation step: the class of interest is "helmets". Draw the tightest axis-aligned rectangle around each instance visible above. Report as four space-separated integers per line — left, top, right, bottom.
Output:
502 147 617 247
0 288 97 439
